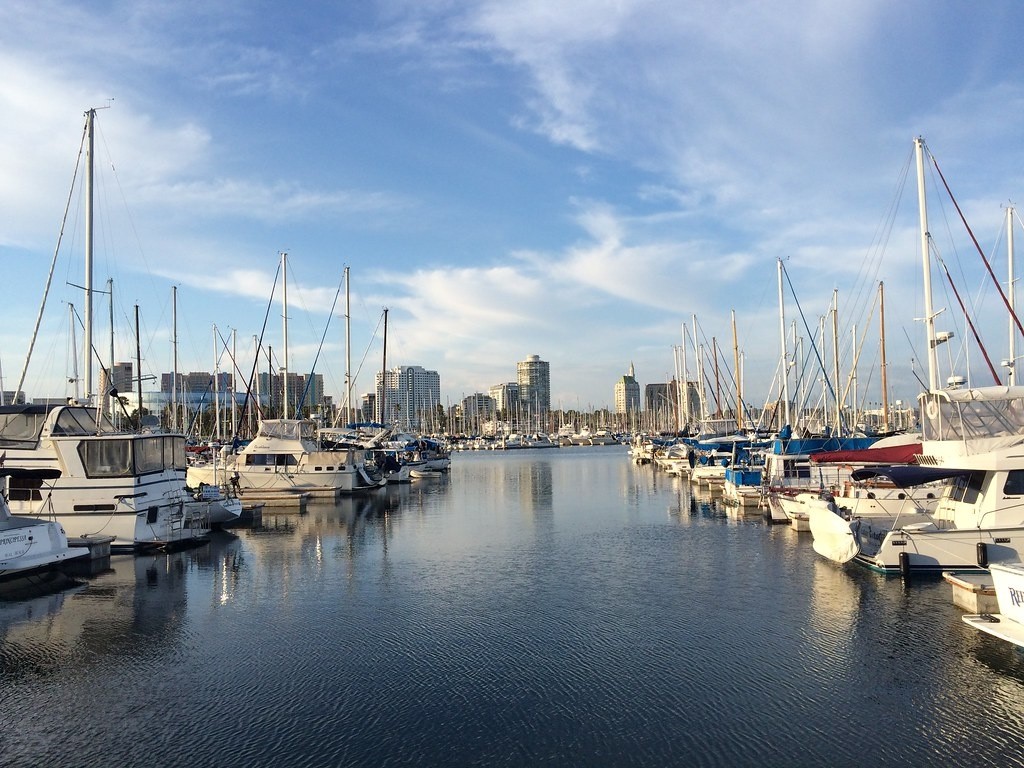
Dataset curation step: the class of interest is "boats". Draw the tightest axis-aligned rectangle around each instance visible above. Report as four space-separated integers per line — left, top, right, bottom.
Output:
0 373 213 553
0 465 92 584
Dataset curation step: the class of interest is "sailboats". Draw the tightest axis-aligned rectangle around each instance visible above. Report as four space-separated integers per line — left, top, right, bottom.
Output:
55 128 1024 659
8 98 243 528
